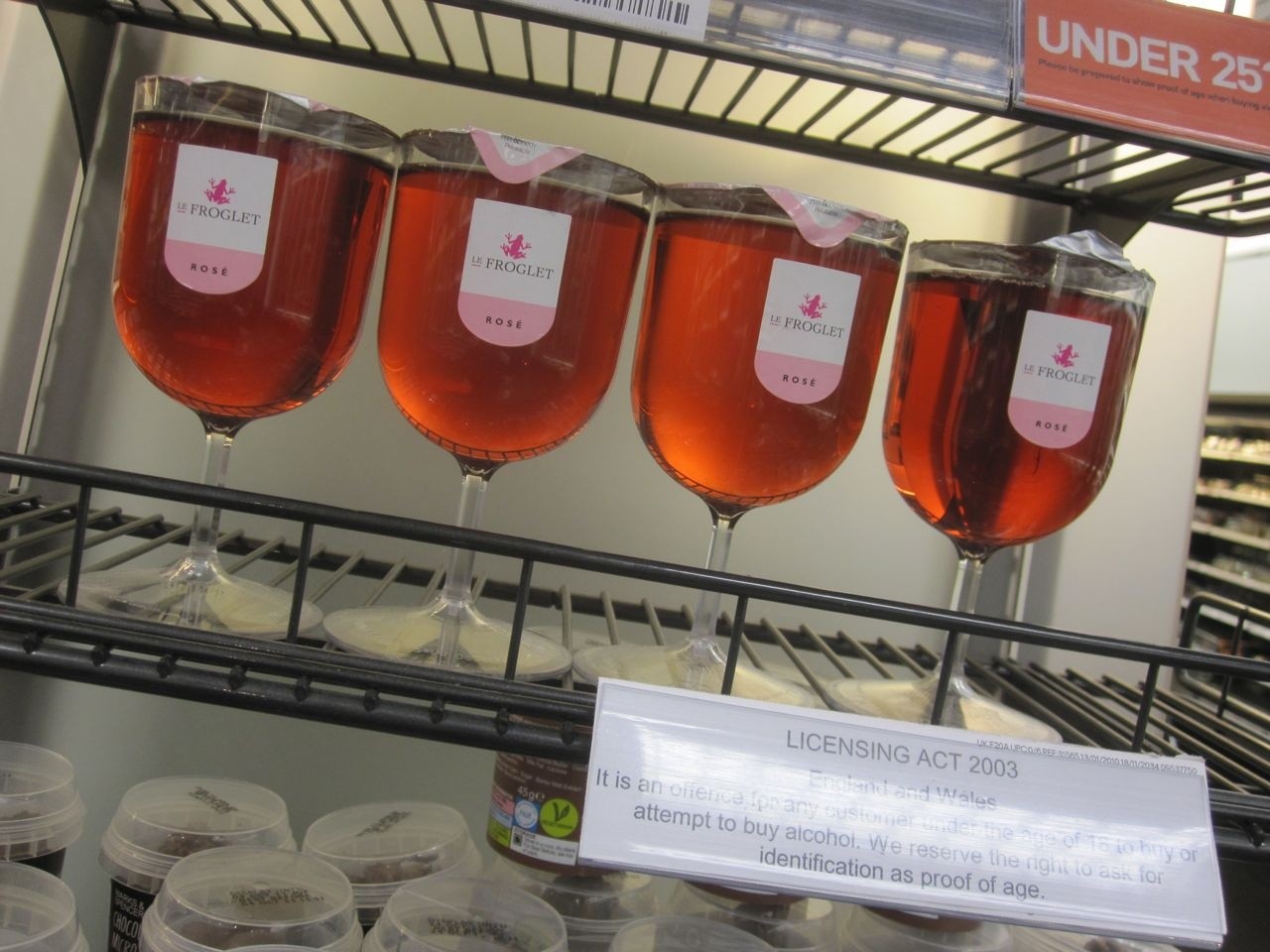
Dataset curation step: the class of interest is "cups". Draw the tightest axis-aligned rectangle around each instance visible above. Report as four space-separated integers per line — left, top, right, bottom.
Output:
0 627 1182 952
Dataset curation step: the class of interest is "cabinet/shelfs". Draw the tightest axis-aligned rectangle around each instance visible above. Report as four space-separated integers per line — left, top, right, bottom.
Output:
1181 414 1270 639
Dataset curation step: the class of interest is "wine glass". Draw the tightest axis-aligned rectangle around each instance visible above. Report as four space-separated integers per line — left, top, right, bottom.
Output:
57 74 400 645
826 238 1156 745
322 128 659 682
571 184 911 707
